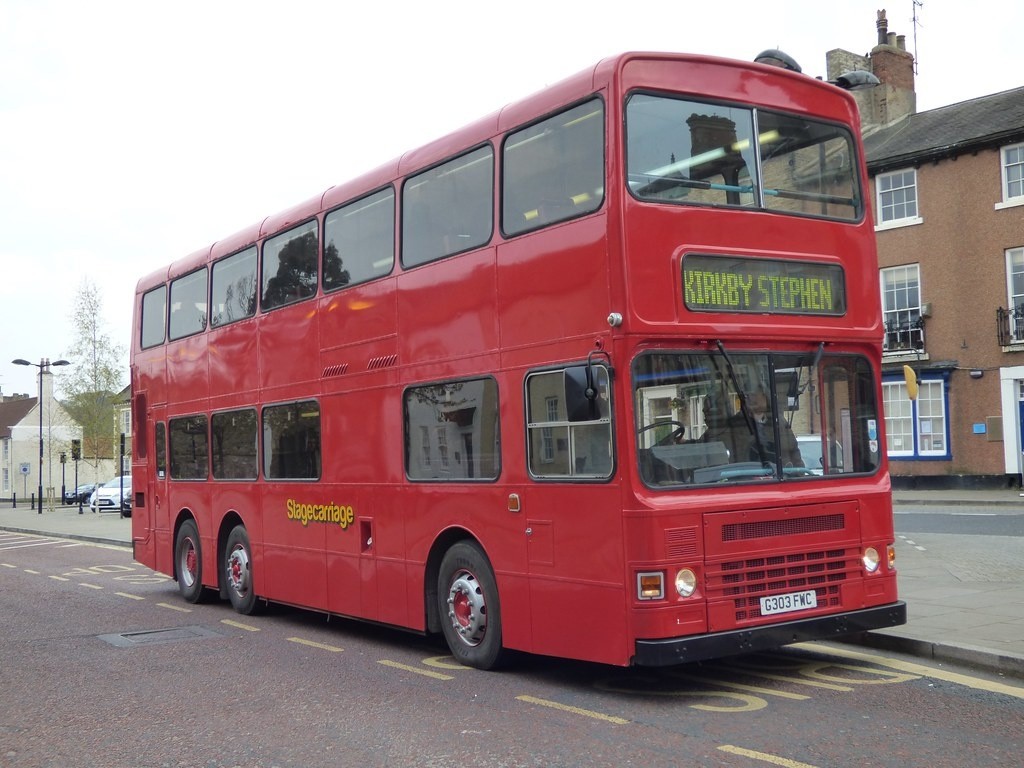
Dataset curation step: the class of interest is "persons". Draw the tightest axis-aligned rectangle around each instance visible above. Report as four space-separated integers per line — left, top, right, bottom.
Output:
724 378 806 476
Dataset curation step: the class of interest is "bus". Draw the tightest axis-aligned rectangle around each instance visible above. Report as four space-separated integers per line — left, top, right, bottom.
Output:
126 47 922 678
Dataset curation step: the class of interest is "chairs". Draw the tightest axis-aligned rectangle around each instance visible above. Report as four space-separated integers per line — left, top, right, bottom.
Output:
444 234 465 252
537 196 575 216
503 207 527 233
170 420 259 480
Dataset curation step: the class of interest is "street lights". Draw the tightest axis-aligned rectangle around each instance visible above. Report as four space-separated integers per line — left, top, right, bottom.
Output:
11 356 70 514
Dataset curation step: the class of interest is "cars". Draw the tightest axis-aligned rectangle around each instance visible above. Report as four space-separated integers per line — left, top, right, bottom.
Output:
123 489 133 519
65 482 106 505
89 475 132 512
